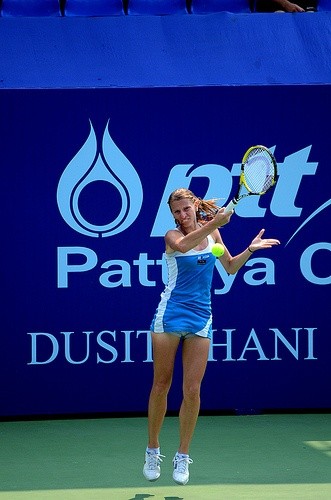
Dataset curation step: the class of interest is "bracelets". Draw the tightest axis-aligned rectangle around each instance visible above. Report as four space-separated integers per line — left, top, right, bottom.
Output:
248 246 254 253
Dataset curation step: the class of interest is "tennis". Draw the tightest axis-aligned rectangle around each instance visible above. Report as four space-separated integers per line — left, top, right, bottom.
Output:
210 242 225 258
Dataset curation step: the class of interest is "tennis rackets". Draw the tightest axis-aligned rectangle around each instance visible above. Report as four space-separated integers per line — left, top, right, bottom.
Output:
219 145 278 215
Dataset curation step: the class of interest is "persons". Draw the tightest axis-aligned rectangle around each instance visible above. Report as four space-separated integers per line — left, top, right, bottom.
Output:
143 188 280 485
264 0 317 13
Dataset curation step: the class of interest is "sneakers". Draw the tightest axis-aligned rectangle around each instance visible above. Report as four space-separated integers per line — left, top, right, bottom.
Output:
172 451 193 485
143 446 166 481
